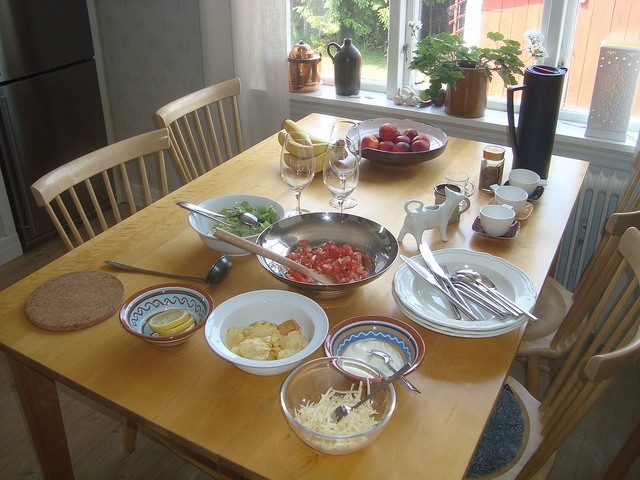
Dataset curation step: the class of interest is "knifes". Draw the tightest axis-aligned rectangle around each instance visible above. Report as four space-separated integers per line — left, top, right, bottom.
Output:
401 244 473 321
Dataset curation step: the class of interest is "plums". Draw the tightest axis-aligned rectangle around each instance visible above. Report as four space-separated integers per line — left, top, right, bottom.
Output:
373 133 379 140
396 131 401 135
379 123 397 142
361 135 380 149
380 142 393 151
393 135 411 145
392 141 411 152
411 135 428 144
402 129 418 141
410 139 429 152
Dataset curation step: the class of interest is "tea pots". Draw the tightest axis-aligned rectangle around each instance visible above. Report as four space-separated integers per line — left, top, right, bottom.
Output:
507 66 568 179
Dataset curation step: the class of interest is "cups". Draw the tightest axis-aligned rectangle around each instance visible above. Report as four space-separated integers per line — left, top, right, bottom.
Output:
509 168 548 197
480 204 515 236
495 187 528 216
434 183 471 223
445 172 476 196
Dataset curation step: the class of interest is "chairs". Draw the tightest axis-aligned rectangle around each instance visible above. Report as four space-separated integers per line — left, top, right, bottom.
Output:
28 128 174 452
517 149 639 399
461 223 640 479
153 79 247 186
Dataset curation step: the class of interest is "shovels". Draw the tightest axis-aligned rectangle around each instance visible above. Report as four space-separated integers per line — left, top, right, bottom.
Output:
211 226 340 287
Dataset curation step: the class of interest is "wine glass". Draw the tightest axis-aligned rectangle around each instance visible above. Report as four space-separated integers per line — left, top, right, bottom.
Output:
327 120 359 208
324 137 360 212
279 131 316 218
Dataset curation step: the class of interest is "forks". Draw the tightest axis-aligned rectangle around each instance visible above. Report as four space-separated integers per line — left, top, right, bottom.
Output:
326 363 412 423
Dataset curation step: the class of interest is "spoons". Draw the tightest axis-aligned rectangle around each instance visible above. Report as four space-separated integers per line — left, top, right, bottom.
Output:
103 253 233 283
364 349 421 395
452 269 535 325
176 199 257 228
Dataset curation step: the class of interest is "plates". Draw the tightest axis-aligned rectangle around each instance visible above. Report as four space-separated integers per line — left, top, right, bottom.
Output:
487 198 534 221
390 248 538 338
502 180 544 201
344 117 449 168
472 215 519 241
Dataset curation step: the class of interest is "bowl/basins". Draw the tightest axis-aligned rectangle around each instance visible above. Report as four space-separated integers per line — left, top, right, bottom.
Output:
205 288 329 376
255 212 398 300
280 357 396 455
187 194 285 258
323 315 426 386
118 282 214 347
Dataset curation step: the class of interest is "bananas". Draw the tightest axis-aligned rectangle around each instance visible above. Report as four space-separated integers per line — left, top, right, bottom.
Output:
278 118 348 174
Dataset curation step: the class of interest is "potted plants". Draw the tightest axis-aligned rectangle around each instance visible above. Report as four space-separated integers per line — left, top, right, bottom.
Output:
401 18 549 117
